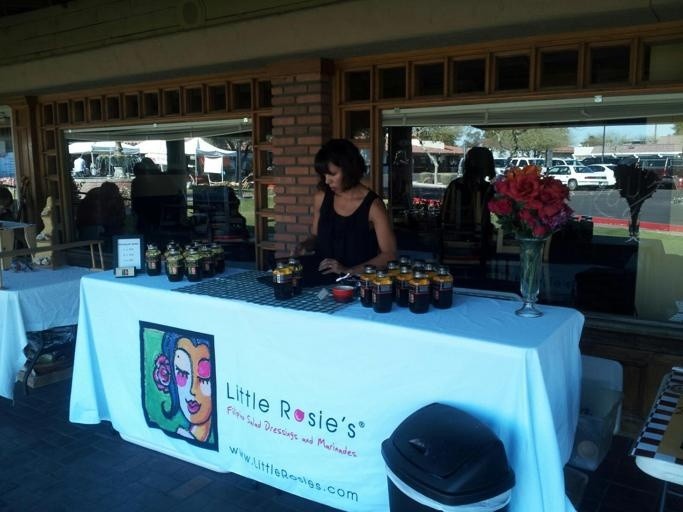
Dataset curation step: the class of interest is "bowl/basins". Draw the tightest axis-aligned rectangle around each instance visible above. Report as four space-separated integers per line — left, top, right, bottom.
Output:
330 286 355 302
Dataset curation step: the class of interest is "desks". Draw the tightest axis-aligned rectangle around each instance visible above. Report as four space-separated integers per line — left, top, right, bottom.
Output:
1 261 103 404
494 234 664 316
71 257 587 511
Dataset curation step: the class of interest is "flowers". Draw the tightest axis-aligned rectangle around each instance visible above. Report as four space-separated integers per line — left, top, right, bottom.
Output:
484 164 575 238
609 160 660 214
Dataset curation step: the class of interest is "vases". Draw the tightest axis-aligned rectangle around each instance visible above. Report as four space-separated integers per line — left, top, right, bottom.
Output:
629 207 639 234
514 237 545 319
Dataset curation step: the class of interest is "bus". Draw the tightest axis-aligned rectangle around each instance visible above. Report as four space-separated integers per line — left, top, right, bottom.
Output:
403 143 467 185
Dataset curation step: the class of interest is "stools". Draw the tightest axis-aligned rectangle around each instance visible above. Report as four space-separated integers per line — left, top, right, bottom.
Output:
630 366 682 512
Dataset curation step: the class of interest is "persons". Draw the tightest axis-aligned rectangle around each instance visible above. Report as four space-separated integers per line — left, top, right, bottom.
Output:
128 156 188 245
77 181 126 250
430 145 495 285
72 153 88 179
289 138 397 276
0 186 14 222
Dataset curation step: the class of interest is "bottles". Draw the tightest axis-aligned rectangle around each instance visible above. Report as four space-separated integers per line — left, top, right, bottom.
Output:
392 205 441 230
272 255 303 303
144 239 225 282
359 255 454 316
568 214 595 243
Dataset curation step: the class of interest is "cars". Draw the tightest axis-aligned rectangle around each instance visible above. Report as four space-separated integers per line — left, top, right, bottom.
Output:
483 150 682 194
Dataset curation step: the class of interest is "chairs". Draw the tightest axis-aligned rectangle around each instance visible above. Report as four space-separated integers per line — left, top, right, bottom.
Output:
437 221 484 286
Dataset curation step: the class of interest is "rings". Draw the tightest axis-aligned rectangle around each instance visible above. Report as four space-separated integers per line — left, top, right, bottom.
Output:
328 262 332 266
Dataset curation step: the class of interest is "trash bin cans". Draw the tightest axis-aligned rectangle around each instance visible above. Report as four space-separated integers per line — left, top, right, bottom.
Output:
381 402 515 512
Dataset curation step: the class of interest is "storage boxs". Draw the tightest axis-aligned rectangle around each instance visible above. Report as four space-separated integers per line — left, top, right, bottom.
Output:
566 381 626 474
